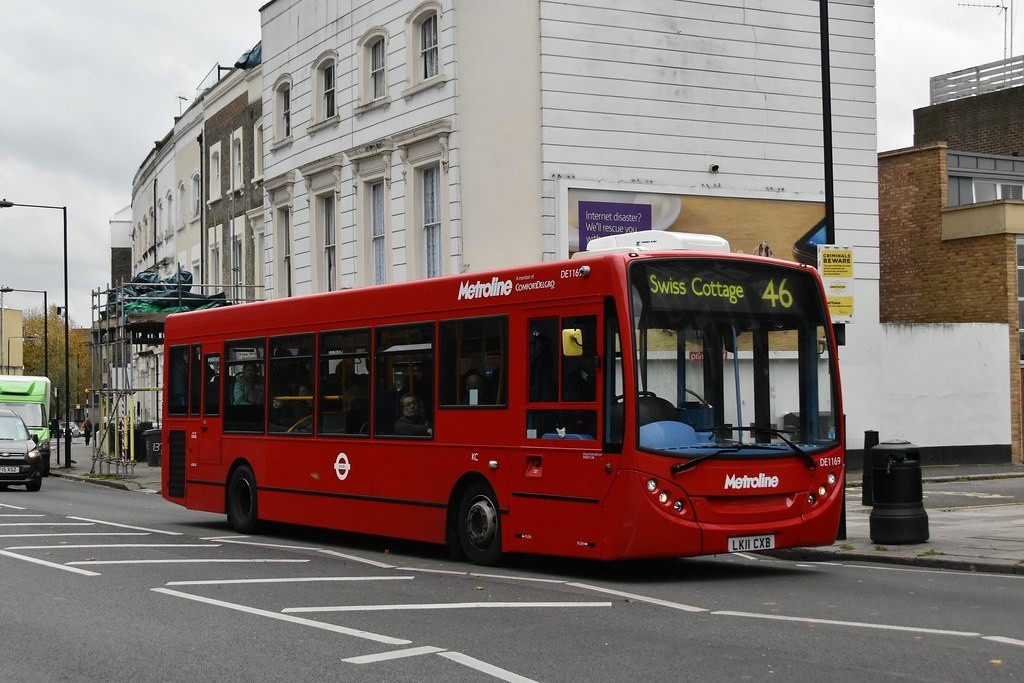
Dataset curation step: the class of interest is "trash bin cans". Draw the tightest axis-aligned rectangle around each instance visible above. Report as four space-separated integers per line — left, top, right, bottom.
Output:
870 439 929 545
143 429 161 467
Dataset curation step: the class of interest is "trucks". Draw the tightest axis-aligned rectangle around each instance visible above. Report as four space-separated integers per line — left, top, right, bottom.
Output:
0 375 58 476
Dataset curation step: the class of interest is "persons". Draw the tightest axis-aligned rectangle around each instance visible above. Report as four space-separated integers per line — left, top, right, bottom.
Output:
82 418 92 446
827 422 835 440
234 363 265 406
753 240 773 258
269 347 435 437
182 345 213 389
445 348 486 406
564 350 618 424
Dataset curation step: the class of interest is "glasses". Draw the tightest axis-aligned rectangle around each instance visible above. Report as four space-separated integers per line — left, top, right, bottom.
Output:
403 402 419 407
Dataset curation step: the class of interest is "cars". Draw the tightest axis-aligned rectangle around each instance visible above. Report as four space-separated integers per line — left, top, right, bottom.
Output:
48 422 92 438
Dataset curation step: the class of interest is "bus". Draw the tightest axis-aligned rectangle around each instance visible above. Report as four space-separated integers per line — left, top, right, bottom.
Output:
162 230 846 565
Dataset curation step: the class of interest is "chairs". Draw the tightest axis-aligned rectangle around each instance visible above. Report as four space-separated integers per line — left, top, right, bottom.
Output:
206 360 371 433
614 392 676 435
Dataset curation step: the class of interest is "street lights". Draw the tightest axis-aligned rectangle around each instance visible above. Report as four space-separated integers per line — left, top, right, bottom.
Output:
0 286 48 377
0 198 73 468
8 334 35 374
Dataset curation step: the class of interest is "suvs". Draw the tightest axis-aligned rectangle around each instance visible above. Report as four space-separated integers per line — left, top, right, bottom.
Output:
0 410 43 491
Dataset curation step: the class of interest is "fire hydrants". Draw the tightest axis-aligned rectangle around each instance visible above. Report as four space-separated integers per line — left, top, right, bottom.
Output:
862 430 879 505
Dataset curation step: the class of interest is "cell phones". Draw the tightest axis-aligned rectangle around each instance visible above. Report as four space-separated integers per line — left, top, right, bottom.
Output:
793 217 826 270
